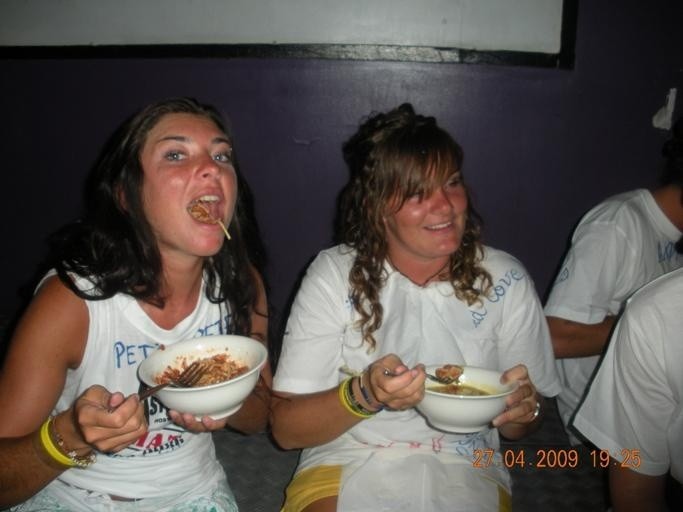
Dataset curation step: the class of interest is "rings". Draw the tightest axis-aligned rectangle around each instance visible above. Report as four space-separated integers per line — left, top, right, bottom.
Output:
533 409 539 417
535 401 540 409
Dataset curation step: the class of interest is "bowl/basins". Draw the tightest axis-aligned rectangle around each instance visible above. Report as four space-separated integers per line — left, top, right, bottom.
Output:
416 366 520 435
137 334 269 421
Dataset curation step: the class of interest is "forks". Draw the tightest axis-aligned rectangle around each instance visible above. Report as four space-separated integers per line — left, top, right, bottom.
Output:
382 369 463 384
109 362 211 411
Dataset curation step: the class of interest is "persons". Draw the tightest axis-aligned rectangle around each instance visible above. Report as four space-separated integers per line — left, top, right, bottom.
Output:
573 266 683 512
269 102 563 512
545 176 683 446
0 96 272 512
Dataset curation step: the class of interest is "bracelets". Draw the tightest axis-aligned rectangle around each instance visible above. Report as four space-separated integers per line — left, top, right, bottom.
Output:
40 415 96 468
338 375 385 419
